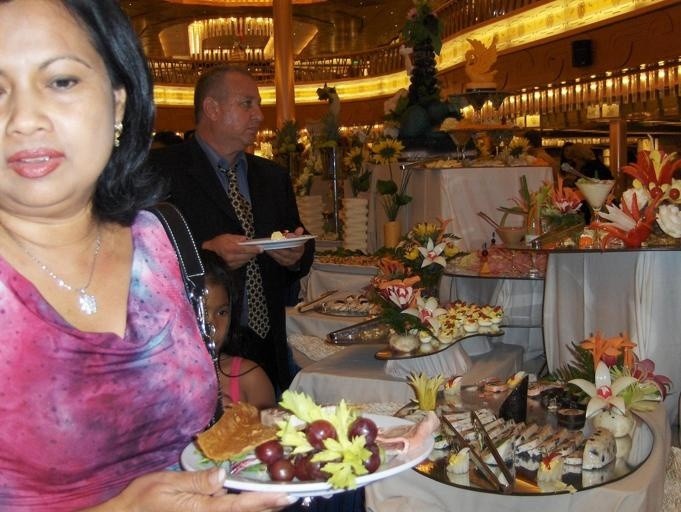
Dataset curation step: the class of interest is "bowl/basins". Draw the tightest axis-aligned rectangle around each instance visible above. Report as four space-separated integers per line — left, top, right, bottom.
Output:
498 225 528 245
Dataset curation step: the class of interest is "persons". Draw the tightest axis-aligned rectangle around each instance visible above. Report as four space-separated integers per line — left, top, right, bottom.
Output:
0 1 303 511
188 252 276 411
519 122 614 224
145 64 316 392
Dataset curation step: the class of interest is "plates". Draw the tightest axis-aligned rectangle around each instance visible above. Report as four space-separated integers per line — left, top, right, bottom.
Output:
180 409 438 493
238 233 318 251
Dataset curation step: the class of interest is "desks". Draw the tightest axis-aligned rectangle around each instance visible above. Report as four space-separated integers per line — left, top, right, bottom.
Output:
362 375 679 510
289 332 527 417
281 142 680 340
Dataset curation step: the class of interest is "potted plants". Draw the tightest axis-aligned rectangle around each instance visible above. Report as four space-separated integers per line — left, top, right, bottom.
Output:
342 141 415 248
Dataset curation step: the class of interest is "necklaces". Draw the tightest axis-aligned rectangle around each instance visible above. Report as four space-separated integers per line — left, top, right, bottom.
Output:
13 224 107 319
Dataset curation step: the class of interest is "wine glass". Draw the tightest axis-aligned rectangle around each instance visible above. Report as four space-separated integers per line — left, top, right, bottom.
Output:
449 131 473 166
575 179 617 247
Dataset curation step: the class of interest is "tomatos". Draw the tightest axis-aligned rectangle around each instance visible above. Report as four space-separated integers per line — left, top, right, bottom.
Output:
651 188 661 199
670 189 679 199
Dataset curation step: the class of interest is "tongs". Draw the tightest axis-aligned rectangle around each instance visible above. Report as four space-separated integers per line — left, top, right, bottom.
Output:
400 153 448 171
298 288 340 312
439 409 516 495
327 315 389 343
531 220 586 247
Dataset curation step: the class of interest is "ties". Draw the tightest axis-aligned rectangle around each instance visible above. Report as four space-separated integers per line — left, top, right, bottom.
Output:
220 164 271 339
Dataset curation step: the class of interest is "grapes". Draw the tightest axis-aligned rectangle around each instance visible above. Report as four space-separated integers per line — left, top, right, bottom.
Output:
255 417 380 482
